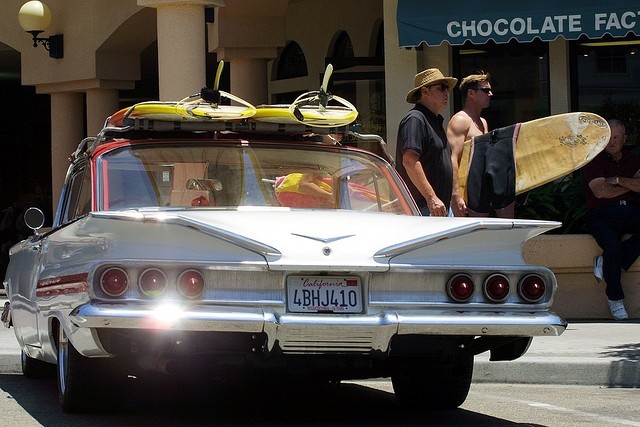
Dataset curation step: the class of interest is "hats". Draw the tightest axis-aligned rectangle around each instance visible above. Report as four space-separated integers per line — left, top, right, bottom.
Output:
405 68 458 103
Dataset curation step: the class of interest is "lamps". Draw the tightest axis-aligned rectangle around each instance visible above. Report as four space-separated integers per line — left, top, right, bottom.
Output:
17 0 64 59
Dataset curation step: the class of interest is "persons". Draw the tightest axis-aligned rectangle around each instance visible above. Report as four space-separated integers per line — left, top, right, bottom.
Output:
445 73 493 217
298 134 342 204
581 119 640 321
395 67 459 216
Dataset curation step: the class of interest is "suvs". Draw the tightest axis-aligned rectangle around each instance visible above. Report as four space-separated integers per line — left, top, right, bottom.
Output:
0 60 568 416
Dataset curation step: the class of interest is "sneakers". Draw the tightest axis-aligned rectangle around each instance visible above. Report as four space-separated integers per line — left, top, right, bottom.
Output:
593 255 604 282
607 297 629 321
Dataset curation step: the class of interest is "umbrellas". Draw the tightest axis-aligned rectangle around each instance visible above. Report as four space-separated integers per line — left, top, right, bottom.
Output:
262 173 391 209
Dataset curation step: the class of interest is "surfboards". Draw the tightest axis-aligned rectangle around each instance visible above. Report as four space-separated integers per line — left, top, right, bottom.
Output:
252 63 359 128
274 172 397 211
107 59 257 126
458 111 612 208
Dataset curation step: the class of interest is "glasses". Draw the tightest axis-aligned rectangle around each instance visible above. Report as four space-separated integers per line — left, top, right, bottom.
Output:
480 88 492 93
425 83 449 91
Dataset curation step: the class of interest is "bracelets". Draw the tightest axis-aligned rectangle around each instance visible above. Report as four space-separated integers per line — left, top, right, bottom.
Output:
615 177 620 184
